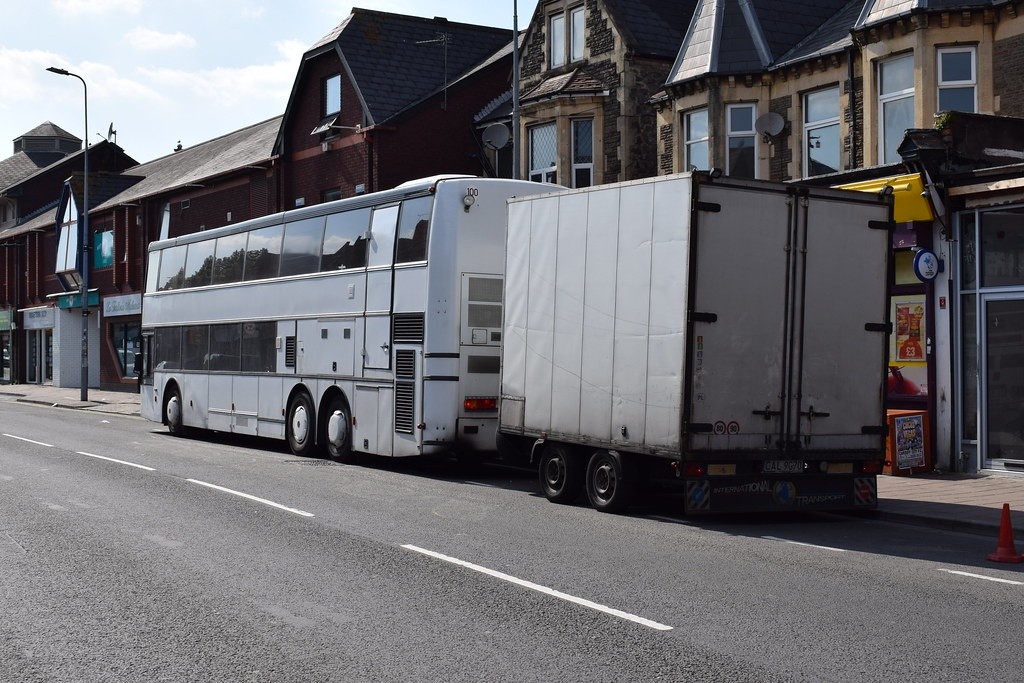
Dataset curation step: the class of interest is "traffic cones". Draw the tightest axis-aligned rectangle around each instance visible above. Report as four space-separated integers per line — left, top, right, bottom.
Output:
986 501 1024 563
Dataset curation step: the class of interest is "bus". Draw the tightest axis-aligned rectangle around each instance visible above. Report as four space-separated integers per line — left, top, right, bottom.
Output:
132 172 575 462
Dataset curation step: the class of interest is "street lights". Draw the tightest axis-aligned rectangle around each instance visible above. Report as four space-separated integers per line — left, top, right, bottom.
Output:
47 65 89 401
